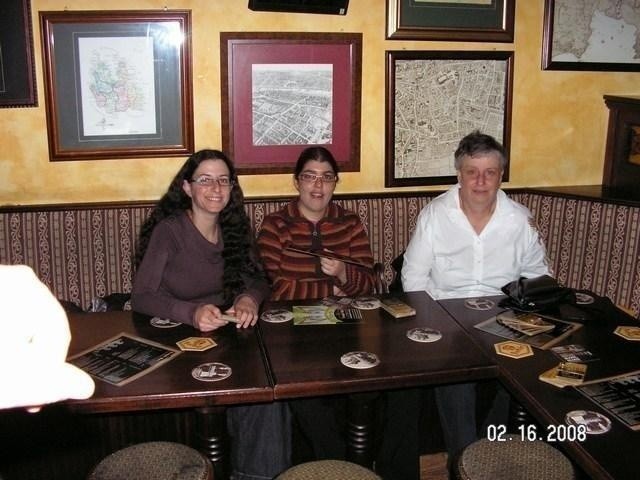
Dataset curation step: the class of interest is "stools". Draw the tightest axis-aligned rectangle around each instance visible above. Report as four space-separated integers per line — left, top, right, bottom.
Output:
273 461 379 480
90 443 209 478
460 436 574 480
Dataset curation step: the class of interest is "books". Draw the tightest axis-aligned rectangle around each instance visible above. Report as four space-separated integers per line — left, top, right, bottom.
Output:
474 307 584 352
66 333 185 387
380 298 416 319
573 371 640 430
551 360 588 388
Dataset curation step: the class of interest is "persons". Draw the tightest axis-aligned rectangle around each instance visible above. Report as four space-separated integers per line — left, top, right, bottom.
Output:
257 146 382 457
400 131 556 455
129 148 290 480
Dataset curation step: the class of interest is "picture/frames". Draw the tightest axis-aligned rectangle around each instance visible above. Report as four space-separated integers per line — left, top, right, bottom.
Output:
220 32 363 175
39 11 195 162
0 0 37 108
540 0 640 72
248 0 349 16
385 0 516 44
385 51 515 187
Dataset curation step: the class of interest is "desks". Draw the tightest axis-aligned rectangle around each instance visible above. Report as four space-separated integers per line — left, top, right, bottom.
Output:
61 313 274 480
437 291 640 480
256 291 499 469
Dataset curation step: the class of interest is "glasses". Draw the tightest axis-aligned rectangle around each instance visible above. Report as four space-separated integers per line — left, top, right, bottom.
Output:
190 174 236 187
461 165 501 180
298 172 339 183
